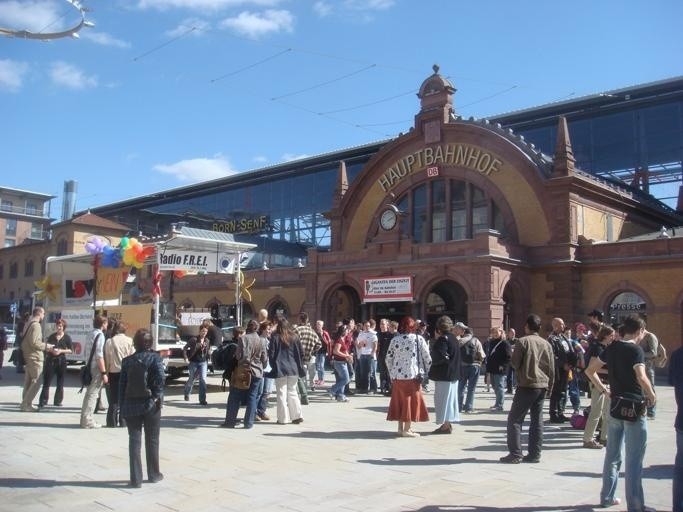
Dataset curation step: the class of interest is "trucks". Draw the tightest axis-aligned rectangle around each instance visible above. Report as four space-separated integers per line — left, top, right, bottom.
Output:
9 234 258 373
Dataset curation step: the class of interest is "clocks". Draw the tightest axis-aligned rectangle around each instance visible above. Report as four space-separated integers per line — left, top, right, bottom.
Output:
380 208 397 231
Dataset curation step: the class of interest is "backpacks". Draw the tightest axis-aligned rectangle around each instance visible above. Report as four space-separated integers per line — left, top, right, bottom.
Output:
654 344 667 369
123 356 153 401
212 344 232 370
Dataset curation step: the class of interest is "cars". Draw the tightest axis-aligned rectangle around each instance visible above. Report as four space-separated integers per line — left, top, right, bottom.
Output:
5 330 16 347
158 324 219 384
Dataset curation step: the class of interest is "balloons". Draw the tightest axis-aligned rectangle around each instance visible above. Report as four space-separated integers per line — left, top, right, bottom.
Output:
84 236 155 269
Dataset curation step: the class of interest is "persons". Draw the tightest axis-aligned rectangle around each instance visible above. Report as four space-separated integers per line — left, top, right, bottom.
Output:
668 349 682 511
80 315 165 488
182 308 658 464
0 326 7 380
19 306 53 413
584 313 658 510
37 318 73 407
127 278 151 305
13 311 30 374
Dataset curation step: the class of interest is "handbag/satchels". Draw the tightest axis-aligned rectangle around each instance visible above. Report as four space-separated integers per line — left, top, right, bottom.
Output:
417 372 424 385
230 366 252 391
608 392 644 422
570 410 586 429
429 352 449 366
298 377 308 406
17 348 24 365
81 366 92 386
459 342 477 362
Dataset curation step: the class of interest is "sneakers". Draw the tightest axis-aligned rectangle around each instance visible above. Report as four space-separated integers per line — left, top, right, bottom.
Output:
397 424 452 436
20 401 64 413
501 455 522 463
584 436 606 449
127 482 142 489
460 403 504 412
183 393 207 404
643 506 655 512
551 410 580 423
328 390 376 403
83 421 102 429
523 456 539 463
311 381 324 390
148 474 163 483
602 499 621 507
222 413 304 427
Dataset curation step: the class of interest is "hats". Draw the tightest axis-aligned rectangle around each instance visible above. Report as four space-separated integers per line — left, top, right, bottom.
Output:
454 322 469 329
420 322 430 326
588 309 603 316
